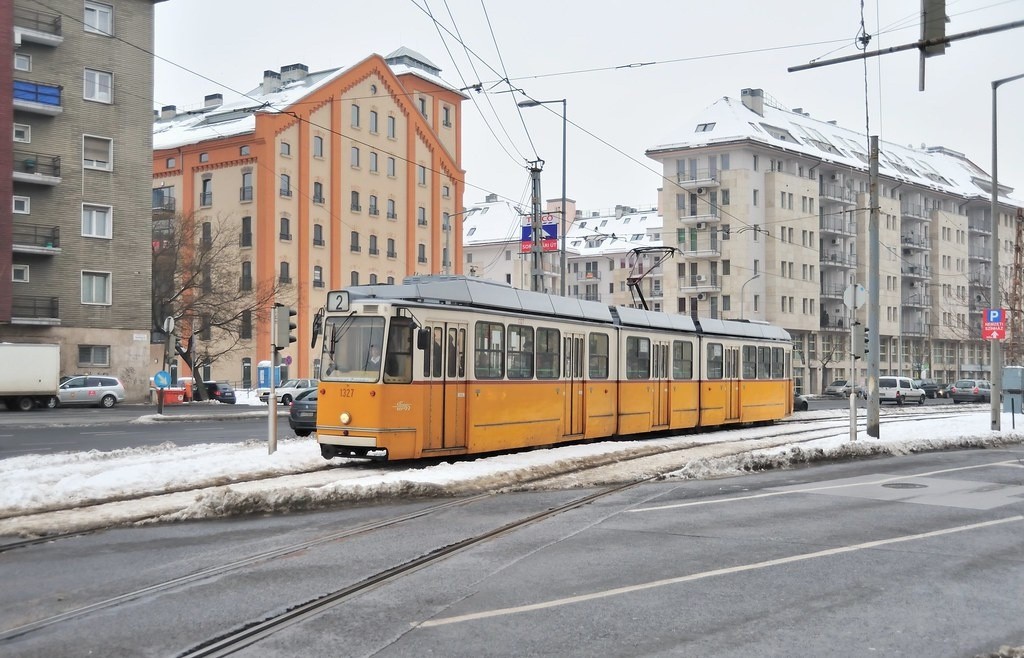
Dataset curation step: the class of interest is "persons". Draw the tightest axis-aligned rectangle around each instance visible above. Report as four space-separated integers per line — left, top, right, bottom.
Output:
516 342 534 369
367 345 390 374
433 333 455 377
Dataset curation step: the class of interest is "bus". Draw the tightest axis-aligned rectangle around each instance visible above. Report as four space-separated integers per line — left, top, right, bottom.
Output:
312 274 794 461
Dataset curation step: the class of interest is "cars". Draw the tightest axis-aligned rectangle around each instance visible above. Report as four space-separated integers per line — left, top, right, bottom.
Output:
183 381 236 404
920 384 939 399
878 376 925 406
951 379 1002 403
824 380 862 397
938 384 952 398
260 378 319 405
289 389 317 437
45 375 125 408
177 377 196 387
794 391 808 411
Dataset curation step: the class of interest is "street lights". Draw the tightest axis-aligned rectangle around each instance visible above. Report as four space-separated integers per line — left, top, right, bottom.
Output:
898 293 918 376
518 98 567 295
446 208 481 275
741 275 760 320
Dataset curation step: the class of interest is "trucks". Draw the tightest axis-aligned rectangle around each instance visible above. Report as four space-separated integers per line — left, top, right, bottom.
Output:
0 343 60 410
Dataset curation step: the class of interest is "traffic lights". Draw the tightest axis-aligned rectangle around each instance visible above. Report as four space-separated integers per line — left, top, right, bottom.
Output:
289 310 297 343
